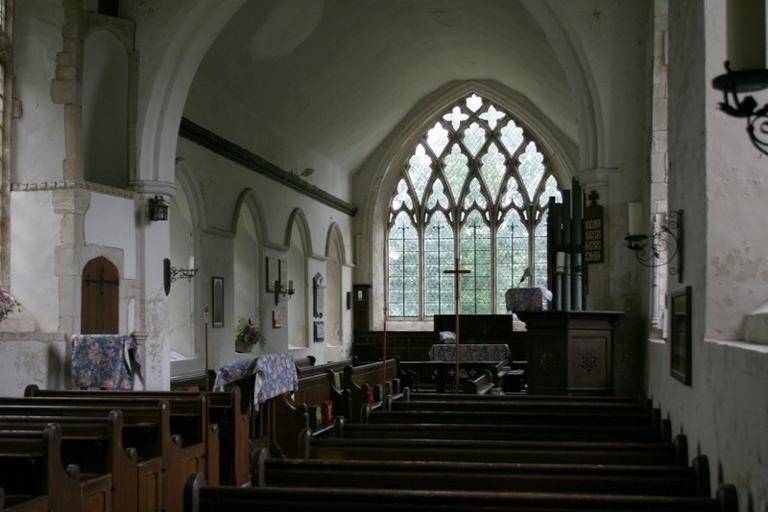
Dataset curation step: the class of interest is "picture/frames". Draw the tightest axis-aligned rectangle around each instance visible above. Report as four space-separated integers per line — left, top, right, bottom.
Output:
211 276 225 329
665 285 694 387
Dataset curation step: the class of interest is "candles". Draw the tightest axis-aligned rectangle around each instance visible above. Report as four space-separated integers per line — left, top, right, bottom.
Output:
289 280 293 291
627 200 644 235
358 290 362 301
188 255 195 270
727 1 765 72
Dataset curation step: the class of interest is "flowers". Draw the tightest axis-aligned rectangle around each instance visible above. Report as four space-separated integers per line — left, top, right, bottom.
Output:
234 317 264 343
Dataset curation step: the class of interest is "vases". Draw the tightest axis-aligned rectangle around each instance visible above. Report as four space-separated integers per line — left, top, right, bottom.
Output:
234 344 254 353
